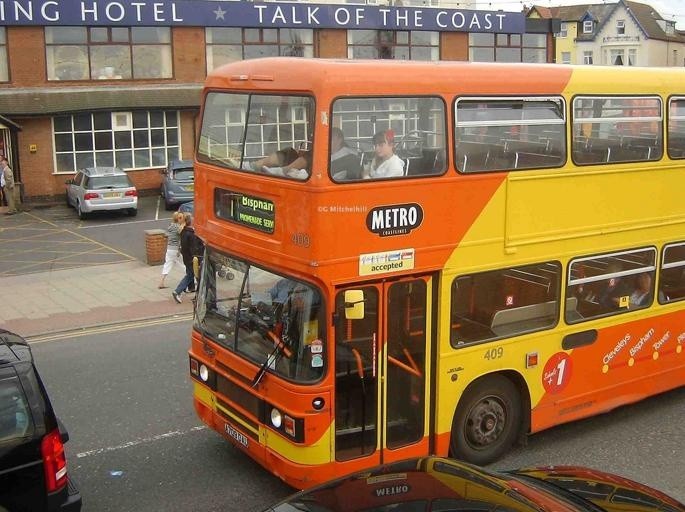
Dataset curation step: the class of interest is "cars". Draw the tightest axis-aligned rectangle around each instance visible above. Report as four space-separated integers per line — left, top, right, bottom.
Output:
262 454 685 512
0 327 79 511
160 160 194 211
65 167 138 220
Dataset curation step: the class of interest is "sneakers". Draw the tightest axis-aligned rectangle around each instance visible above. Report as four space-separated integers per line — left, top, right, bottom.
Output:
184 288 196 293
172 291 182 303
262 164 287 177
230 159 255 172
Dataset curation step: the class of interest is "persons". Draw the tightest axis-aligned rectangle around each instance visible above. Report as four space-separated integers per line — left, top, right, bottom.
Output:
1 159 17 215
158 211 207 304
359 130 406 179
597 264 631 308
266 279 319 313
632 269 665 306
0 155 7 207
247 121 314 174
331 127 358 181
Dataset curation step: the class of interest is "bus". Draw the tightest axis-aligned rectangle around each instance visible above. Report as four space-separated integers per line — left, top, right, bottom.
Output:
190 57 684 491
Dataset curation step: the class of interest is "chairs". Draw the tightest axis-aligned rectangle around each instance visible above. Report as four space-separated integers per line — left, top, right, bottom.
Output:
297 140 312 156
